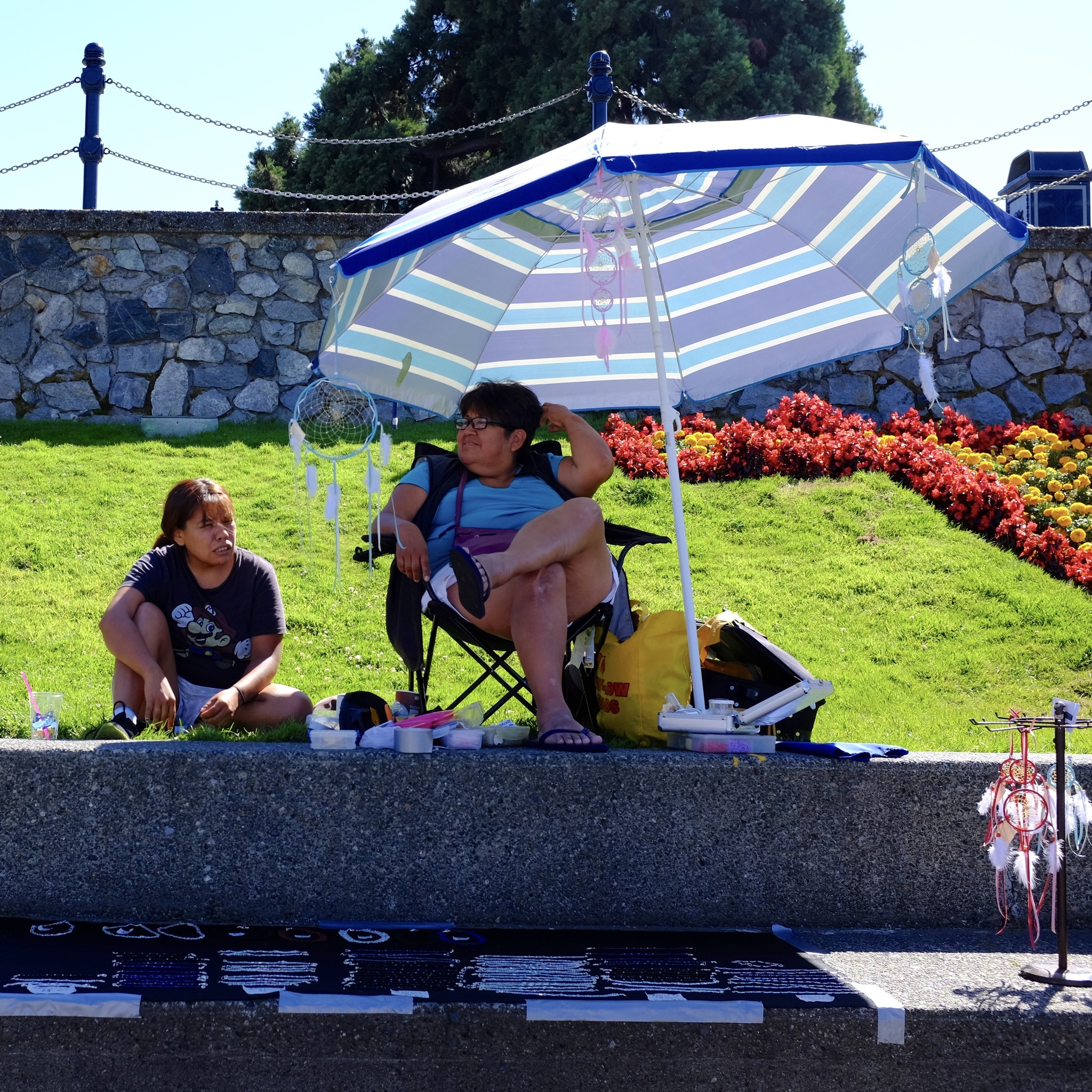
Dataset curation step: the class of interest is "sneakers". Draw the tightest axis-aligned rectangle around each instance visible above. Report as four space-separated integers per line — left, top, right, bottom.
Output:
95 700 140 741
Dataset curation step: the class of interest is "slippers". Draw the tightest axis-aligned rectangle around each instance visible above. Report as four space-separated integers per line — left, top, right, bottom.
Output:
449 546 491 620
533 728 608 753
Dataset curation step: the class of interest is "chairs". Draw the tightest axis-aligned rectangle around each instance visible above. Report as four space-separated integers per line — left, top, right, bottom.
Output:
354 442 673 729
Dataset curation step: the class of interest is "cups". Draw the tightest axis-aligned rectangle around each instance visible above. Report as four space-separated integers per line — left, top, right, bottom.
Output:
709 699 734 716
28 692 64 740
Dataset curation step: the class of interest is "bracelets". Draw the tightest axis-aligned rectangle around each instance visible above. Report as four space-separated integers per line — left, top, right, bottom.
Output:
233 685 245 707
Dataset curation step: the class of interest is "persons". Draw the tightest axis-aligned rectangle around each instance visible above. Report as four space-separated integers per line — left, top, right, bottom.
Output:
372 377 620 754
96 479 313 738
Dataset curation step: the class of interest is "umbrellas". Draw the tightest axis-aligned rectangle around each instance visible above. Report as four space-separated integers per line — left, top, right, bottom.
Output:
317 106 1030 698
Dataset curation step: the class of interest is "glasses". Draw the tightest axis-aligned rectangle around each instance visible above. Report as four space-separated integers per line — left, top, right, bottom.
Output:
454 417 506 430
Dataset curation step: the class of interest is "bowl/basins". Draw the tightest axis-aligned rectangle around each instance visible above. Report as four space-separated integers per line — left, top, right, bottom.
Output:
309 729 358 750
475 726 530 748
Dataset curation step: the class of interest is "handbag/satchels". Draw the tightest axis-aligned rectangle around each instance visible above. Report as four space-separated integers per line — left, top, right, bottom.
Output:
590 597 737 748
